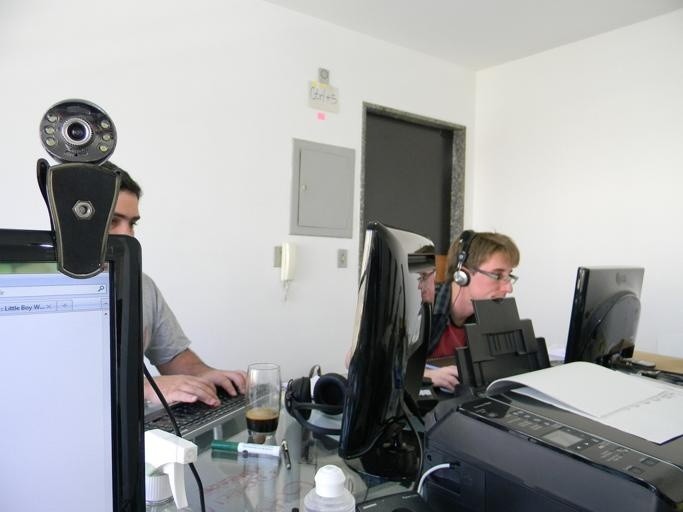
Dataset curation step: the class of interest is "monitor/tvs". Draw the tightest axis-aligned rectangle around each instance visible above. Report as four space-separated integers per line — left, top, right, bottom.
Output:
338 221 436 505
563 265 645 364
0 229 146 512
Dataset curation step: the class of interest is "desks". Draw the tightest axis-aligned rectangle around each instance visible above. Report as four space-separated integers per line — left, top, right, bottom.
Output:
144 403 423 512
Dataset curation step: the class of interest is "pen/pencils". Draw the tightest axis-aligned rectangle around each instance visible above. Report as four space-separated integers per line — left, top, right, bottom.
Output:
282 441 291 469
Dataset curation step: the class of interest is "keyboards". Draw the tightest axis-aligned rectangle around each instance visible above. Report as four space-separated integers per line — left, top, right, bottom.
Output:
143 383 279 440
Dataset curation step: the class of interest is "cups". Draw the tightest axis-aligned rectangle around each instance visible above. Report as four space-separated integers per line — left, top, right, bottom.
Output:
243 364 282 436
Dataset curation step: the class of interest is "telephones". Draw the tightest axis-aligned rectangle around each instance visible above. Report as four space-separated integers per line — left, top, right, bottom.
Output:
281 241 294 282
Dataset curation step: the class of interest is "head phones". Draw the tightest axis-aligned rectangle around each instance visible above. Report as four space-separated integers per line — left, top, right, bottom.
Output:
284 373 349 435
451 231 477 287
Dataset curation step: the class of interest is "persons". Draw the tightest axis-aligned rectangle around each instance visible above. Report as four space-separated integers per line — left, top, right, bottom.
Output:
421 227 520 394
103 161 249 408
413 244 435 322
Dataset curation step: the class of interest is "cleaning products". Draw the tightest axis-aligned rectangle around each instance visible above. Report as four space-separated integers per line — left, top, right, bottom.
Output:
145 429 198 512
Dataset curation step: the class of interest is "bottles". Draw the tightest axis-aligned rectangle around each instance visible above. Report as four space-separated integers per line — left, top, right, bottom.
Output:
303 465 355 512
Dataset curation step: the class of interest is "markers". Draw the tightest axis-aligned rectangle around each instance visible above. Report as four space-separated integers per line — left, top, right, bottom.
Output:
210 441 281 456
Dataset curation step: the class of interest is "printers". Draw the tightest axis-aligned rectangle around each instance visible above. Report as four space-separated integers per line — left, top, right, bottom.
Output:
418 297 683 511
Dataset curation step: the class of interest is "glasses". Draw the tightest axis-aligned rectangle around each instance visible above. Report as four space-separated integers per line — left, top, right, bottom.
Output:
471 267 518 285
